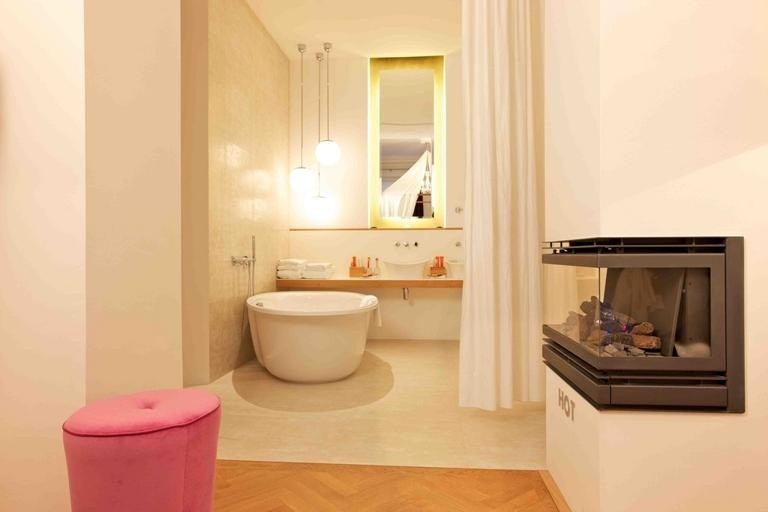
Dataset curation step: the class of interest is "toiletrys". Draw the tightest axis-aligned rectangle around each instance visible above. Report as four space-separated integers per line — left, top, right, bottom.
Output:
372 257 382 275
365 256 371 276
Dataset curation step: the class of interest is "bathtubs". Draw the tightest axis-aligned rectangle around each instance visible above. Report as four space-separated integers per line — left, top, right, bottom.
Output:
245 289 379 384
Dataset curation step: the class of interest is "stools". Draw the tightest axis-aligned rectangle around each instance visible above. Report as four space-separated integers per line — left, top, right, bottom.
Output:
61 390 222 509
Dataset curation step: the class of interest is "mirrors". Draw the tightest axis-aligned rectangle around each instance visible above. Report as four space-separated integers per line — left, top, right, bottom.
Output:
365 53 448 229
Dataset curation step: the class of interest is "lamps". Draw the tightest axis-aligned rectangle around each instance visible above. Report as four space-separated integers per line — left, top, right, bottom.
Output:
289 41 316 188
316 39 344 170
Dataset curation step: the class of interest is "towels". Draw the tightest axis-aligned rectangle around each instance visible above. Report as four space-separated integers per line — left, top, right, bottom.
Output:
276 256 330 282
360 295 383 328
